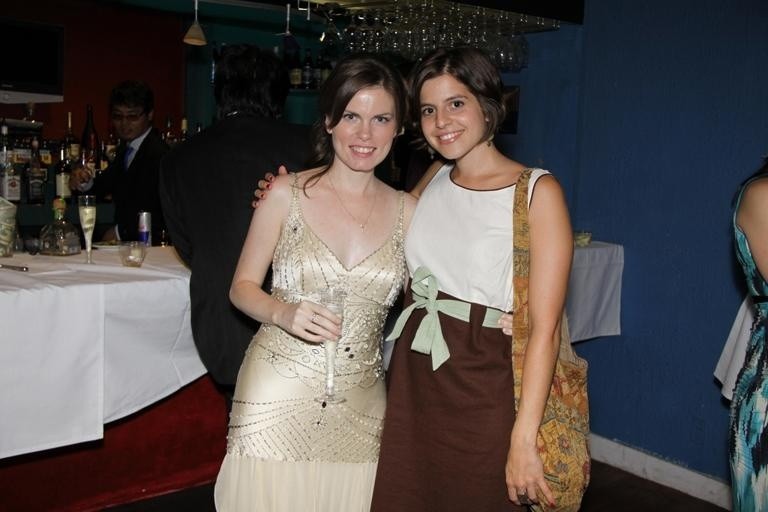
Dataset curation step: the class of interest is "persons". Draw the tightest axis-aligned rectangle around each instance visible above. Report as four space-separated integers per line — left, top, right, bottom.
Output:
69 75 176 243
213 57 417 512
712 174 767 511
251 48 592 512
159 42 305 407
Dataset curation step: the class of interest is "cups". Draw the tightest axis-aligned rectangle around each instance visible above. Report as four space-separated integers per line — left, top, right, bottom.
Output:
118 240 146 268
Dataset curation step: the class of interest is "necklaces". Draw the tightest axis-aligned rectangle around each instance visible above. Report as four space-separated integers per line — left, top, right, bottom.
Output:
326 170 378 234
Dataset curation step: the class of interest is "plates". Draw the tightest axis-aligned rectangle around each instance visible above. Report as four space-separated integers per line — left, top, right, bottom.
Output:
92 241 126 251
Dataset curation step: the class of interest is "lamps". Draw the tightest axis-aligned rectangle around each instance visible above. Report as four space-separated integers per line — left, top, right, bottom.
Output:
182 1 208 47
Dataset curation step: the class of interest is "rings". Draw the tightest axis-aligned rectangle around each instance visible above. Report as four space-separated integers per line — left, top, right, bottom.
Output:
311 314 316 321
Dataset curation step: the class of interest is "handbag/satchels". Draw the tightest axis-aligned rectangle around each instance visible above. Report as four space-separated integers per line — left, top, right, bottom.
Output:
512 352 592 511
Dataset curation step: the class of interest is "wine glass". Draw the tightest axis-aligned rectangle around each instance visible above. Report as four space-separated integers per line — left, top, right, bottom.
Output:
77 195 98 265
314 288 348 406
319 6 530 76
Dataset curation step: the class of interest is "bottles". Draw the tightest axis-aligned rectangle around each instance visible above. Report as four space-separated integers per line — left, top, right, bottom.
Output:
0 103 204 206
38 207 81 256
289 46 330 90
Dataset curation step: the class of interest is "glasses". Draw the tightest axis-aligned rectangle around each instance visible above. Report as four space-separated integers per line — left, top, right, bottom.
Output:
107 106 145 123
